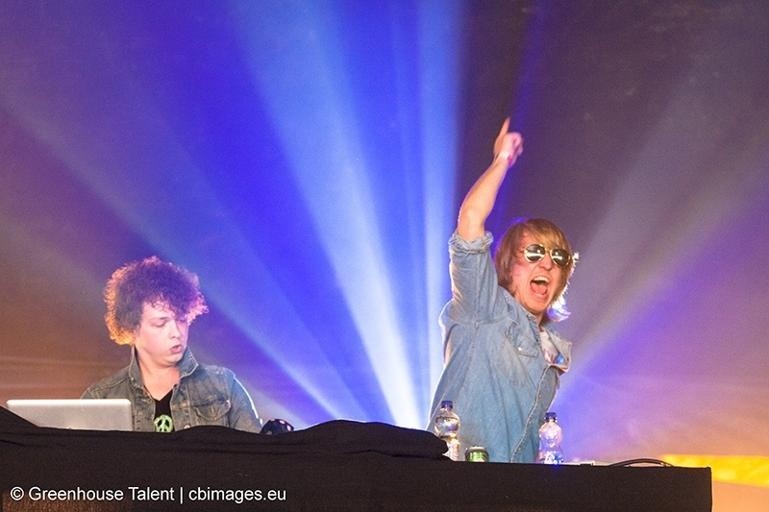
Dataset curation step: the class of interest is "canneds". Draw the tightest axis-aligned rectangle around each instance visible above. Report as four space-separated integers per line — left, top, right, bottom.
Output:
466 447 490 462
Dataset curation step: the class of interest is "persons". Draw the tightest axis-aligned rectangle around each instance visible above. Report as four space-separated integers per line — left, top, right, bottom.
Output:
427 116 574 464
80 255 262 437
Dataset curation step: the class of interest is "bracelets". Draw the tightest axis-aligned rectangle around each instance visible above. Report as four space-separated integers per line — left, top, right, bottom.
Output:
492 151 516 166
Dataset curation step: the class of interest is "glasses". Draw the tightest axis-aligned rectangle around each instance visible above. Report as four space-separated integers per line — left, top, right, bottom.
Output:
524 241 571 269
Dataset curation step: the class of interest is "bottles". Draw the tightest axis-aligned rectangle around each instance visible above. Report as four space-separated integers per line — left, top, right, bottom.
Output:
434 401 463 460
536 411 567 465
